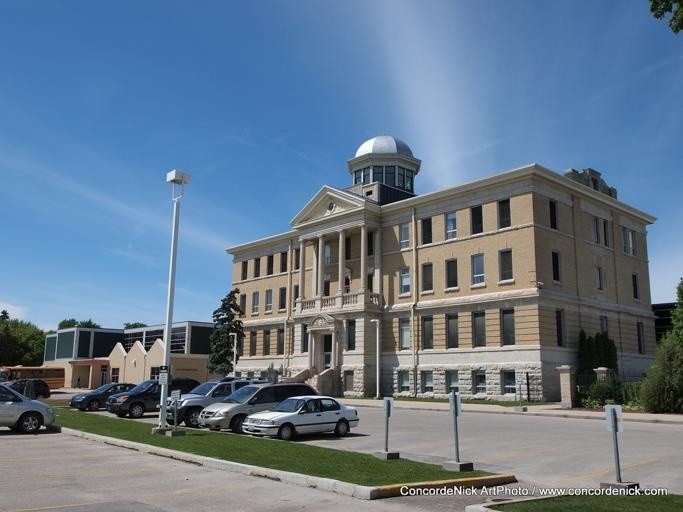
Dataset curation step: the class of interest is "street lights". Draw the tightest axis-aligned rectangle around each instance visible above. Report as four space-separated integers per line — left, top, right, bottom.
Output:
154 162 194 431
369 319 381 401
229 332 236 379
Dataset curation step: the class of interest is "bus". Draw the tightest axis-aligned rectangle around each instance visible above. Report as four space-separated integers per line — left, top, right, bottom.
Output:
0 363 66 391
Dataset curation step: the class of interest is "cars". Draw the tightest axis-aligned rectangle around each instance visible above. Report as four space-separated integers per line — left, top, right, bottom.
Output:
0 381 11 385
67 383 138 413
0 383 55 434
241 396 361 441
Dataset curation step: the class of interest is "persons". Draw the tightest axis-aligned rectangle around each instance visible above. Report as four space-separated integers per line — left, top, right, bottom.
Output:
74 375 82 388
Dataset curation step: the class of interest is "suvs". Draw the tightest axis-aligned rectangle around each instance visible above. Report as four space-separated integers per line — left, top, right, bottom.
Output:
4 379 52 399
104 378 201 418
158 375 267 428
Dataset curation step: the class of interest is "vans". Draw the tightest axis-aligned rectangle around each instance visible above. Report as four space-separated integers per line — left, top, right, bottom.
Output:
197 382 320 433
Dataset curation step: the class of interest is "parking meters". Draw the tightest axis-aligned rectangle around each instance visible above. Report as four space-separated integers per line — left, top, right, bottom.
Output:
516 379 523 408
